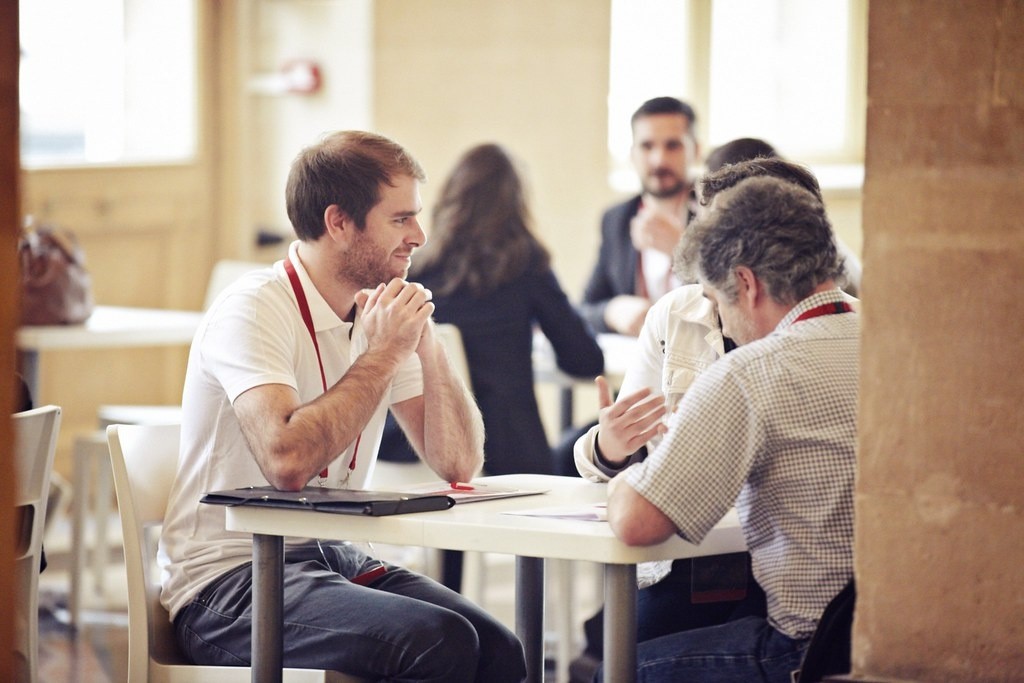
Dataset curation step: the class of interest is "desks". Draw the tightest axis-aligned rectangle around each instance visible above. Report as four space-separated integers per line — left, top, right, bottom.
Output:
13 300 205 406
530 332 639 431
221 468 751 681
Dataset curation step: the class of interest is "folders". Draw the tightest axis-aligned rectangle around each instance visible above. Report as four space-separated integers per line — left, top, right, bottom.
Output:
199 484 457 517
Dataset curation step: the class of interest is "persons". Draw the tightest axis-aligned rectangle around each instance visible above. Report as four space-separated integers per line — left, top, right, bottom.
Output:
703 135 779 170
566 177 862 676
154 130 531 682
574 94 714 335
382 140 613 467
567 154 860 479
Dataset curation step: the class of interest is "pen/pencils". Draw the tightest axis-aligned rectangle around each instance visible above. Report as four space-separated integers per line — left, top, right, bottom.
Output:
450 480 518 493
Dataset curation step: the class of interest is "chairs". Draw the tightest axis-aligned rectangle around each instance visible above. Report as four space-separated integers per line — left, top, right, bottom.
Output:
104 422 367 683
9 404 66 682
66 399 179 630
